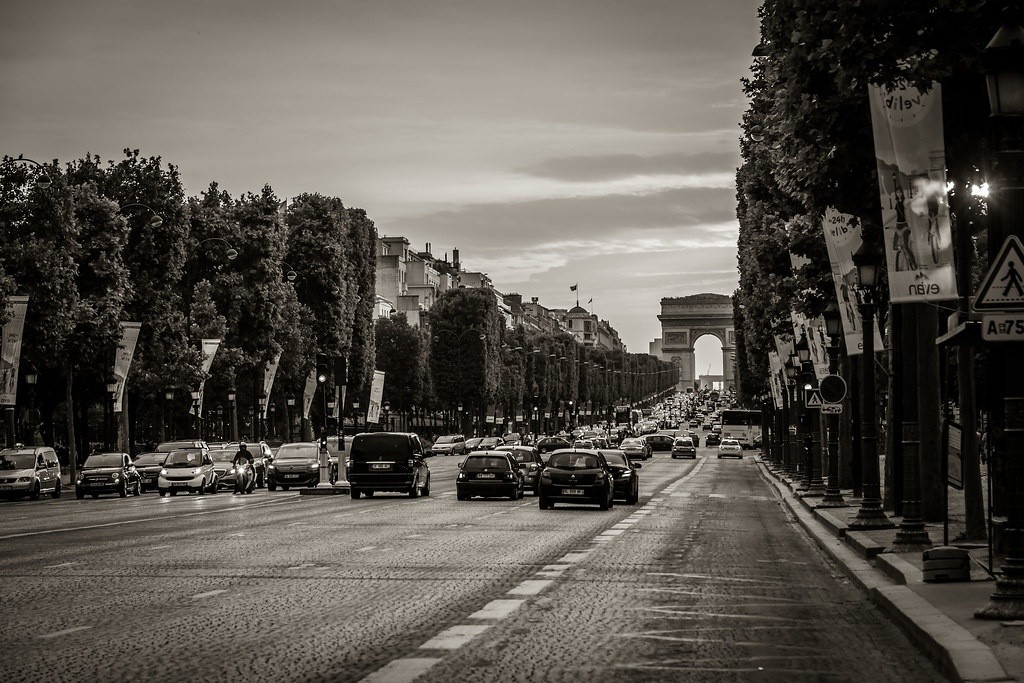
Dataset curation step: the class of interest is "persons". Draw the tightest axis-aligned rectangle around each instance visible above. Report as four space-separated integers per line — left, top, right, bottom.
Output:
232 442 254 494
521 421 575 445
190 452 201 464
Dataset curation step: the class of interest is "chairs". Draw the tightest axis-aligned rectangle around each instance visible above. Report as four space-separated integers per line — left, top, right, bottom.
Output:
585 457 595 467
497 460 506 467
557 456 570 466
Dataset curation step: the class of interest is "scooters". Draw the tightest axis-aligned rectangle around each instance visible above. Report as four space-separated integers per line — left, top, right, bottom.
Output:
229 458 256 494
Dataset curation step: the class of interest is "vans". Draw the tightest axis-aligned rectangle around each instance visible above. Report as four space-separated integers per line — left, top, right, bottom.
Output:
346 430 435 498
0 442 64 500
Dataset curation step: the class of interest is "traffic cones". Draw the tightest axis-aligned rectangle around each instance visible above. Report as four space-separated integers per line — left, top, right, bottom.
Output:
457 399 464 437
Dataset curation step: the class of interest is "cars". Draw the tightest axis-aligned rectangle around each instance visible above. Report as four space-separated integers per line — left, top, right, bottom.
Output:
267 442 337 492
321 434 356 478
536 448 616 510
671 439 697 459
158 446 219 496
717 439 744 460
600 450 642 505
74 451 142 498
429 383 739 496
132 438 276 497
456 451 527 503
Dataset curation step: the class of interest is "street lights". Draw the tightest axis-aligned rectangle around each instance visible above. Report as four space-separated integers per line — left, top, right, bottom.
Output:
190 384 201 439
105 370 119 452
382 398 390 432
227 383 236 441
23 361 39 448
848 227 896 531
969 14 1024 626
759 329 815 492
325 394 335 439
165 380 176 442
814 288 844 509
268 400 277 437
351 396 361 433
257 390 268 442
285 391 299 442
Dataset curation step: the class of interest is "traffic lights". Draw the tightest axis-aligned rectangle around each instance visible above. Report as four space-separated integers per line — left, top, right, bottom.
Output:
315 352 327 385
532 396 540 412
567 400 573 414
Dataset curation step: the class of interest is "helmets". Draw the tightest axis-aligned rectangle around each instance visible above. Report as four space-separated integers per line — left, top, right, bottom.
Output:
239 442 247 446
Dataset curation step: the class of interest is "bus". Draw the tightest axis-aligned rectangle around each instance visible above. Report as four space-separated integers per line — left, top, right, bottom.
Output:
721 408 771 449
